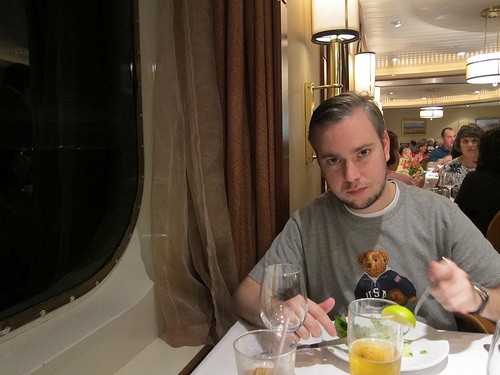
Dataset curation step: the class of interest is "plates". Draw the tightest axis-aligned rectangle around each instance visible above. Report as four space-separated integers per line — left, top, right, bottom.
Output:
322 316 451 372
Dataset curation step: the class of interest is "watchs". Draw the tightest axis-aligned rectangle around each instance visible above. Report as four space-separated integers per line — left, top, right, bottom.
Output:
469 281 489 315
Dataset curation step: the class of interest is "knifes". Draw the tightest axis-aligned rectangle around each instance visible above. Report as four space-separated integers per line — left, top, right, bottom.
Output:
297 337 347 350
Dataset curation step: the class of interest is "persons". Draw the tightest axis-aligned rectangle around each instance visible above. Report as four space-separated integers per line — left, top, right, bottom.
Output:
453 122 500 238
383 130 424 188
398 138 443 158
233 90 500 346
421 125 458 172
439 123 484 196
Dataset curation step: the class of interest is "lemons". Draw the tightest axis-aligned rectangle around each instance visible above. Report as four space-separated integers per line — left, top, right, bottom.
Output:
381 305 416 328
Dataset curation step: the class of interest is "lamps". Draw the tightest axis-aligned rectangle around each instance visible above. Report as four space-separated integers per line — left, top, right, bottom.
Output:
419 87 444 120
466 7 500 86
311 0 360 45
355 51 376 99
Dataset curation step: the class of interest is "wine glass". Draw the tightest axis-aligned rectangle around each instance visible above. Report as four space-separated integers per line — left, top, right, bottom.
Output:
424 162 456 202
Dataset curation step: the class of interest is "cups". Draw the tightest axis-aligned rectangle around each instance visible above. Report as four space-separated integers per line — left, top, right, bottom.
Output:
233 330 297 375
485 320 500 374
258 263 307 333
348 298 403 375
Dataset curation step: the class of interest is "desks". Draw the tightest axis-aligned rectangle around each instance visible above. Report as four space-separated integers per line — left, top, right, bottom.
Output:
191 319 500 375
425 167 439 186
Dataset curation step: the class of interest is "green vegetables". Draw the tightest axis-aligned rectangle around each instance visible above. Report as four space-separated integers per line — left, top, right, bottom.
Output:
334 313 347 338
